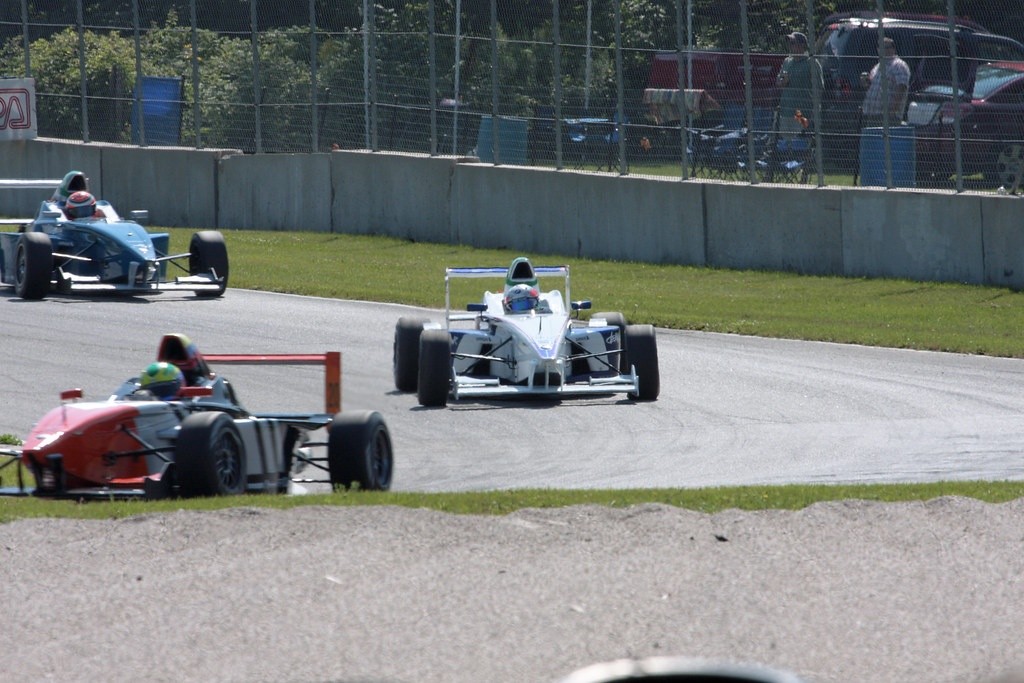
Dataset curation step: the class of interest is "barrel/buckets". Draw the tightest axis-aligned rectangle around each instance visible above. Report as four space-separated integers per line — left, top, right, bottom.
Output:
132 77 182 147
859 127 916 188
477 116 527 165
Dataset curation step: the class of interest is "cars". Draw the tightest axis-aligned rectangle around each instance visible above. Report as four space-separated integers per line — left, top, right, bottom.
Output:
901 61 1024 192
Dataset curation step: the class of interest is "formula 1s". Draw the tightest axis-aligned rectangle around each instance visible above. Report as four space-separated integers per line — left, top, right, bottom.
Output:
0 178 230 302
393 266 662 407
1 333 395 502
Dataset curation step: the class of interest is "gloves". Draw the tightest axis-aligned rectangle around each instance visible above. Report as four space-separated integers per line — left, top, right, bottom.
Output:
134 389 158 400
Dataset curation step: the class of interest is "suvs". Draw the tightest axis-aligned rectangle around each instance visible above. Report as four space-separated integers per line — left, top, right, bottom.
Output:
820 10 1024 169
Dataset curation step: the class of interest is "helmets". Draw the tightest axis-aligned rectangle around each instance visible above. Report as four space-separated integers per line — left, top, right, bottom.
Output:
64 190 96 221
142 363 186 401
502 284 539 315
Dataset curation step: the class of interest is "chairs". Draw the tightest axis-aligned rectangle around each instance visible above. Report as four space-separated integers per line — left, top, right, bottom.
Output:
156 335 211 392
50 170 89 214
504 259 541 303
478 117 918 187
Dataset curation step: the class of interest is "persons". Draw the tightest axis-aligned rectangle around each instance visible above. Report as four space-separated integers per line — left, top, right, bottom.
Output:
133 362 185 400
504 283 540 314
862 35 910 127
775 31 825 138
64 191 106 221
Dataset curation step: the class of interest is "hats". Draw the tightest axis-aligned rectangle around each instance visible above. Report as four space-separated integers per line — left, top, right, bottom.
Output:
785 32 807 44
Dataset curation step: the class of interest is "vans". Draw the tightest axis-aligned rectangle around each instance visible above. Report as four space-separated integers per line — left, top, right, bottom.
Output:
643 48 796 134
